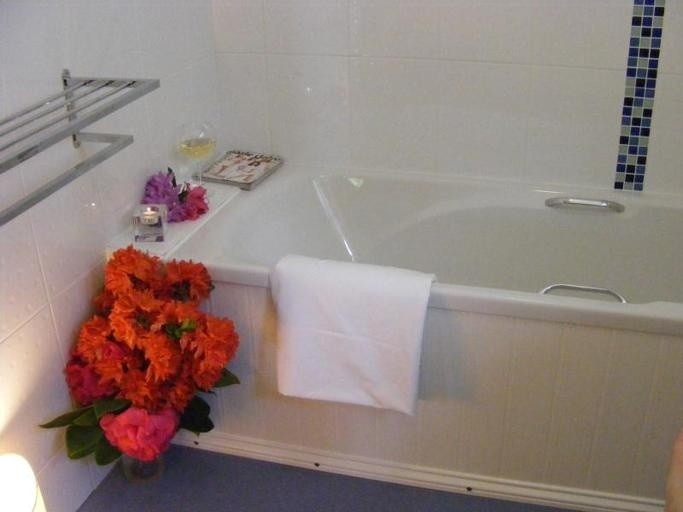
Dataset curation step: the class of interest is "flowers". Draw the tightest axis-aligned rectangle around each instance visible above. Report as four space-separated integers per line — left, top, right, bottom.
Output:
33 243 240 463
141 164 210 224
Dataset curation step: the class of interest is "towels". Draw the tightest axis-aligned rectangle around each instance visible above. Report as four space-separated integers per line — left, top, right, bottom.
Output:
267 254 438 419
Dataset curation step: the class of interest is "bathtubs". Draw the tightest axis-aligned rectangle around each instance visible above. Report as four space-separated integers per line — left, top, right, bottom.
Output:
106 166 683 512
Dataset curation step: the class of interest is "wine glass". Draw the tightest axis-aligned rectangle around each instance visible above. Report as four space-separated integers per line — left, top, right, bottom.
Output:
176 121 216 199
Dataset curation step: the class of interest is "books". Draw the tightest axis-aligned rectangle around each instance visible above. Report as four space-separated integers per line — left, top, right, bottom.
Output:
196 150 283 192
